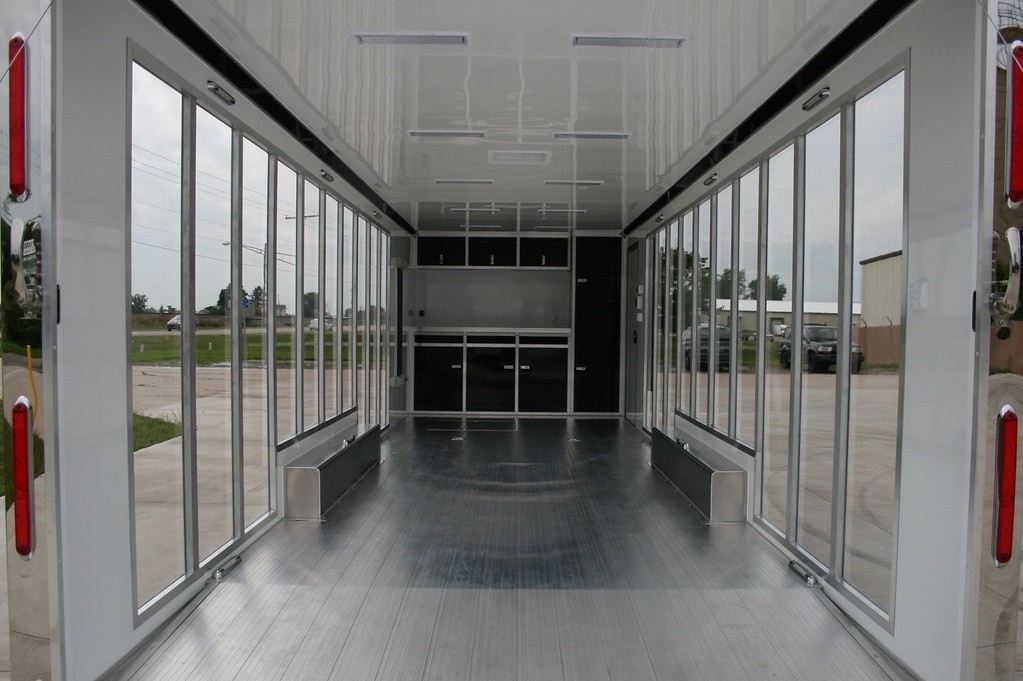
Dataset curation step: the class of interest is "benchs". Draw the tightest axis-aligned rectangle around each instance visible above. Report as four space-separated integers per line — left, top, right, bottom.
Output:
281 423 381 520
652 424 748 525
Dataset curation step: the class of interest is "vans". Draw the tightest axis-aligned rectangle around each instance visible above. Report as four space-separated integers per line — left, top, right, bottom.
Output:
774 325 788 337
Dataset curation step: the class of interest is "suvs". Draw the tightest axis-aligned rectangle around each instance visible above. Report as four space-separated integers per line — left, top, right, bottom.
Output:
682 321 732 371
779 323 867 371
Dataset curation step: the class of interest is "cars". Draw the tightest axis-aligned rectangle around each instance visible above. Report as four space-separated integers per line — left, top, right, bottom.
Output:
309 319 334 332
167 314 200 332
754 329 775 342
